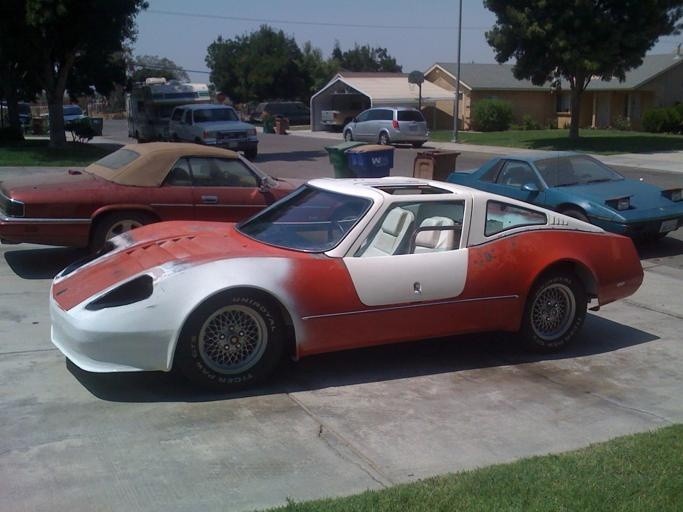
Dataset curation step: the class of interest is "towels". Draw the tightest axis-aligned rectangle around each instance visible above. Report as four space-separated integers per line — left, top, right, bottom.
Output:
31 116 46 133
411 150 460 183
275 117 285 134
324 141 394 177
263 117 274 132
91 118 103 136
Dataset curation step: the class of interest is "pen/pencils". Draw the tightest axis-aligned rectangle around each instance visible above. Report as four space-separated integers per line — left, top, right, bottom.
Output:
344 109 429 148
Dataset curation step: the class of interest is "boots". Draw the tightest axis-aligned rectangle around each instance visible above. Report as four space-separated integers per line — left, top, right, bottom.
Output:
169 103 258 157
252 101 311 127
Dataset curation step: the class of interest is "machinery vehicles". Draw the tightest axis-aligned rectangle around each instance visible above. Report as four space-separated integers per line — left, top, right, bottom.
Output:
50 174 642 393
442 148 682 243
0 142 320 251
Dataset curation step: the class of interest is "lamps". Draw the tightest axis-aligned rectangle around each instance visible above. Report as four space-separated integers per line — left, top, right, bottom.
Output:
173 167 189 181
503 166 533 184
191 175 220 186
547 169 581 188
411 216 455 254
356 207 415 257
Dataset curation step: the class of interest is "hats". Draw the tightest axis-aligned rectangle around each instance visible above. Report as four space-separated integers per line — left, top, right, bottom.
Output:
2 101 89 135
